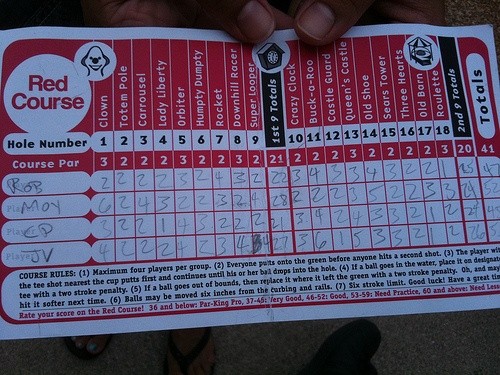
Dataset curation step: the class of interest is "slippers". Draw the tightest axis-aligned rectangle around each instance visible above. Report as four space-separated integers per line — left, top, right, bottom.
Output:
63 334 113 360
163 328 216 375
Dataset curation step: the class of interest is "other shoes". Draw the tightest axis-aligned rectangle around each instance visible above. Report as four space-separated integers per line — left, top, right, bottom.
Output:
300 321 380 375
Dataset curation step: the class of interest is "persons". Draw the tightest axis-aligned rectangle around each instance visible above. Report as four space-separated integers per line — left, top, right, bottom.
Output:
0 0 500 375
